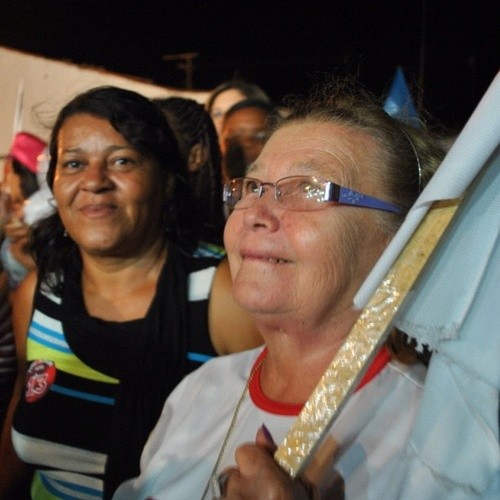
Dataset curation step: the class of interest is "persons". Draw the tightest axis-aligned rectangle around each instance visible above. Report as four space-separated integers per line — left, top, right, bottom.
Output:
0 79 299 500
111 97 447 500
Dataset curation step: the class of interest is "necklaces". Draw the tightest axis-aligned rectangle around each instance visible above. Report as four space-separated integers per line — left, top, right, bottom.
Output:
201 353 265 500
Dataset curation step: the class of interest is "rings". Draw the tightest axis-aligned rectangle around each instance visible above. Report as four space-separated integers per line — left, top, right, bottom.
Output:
210 472 230 500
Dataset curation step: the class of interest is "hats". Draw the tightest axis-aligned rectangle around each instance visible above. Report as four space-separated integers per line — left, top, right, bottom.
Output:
0 132 48 172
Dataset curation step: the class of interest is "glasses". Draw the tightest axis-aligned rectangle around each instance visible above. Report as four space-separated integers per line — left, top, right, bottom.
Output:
223 175 403 215
